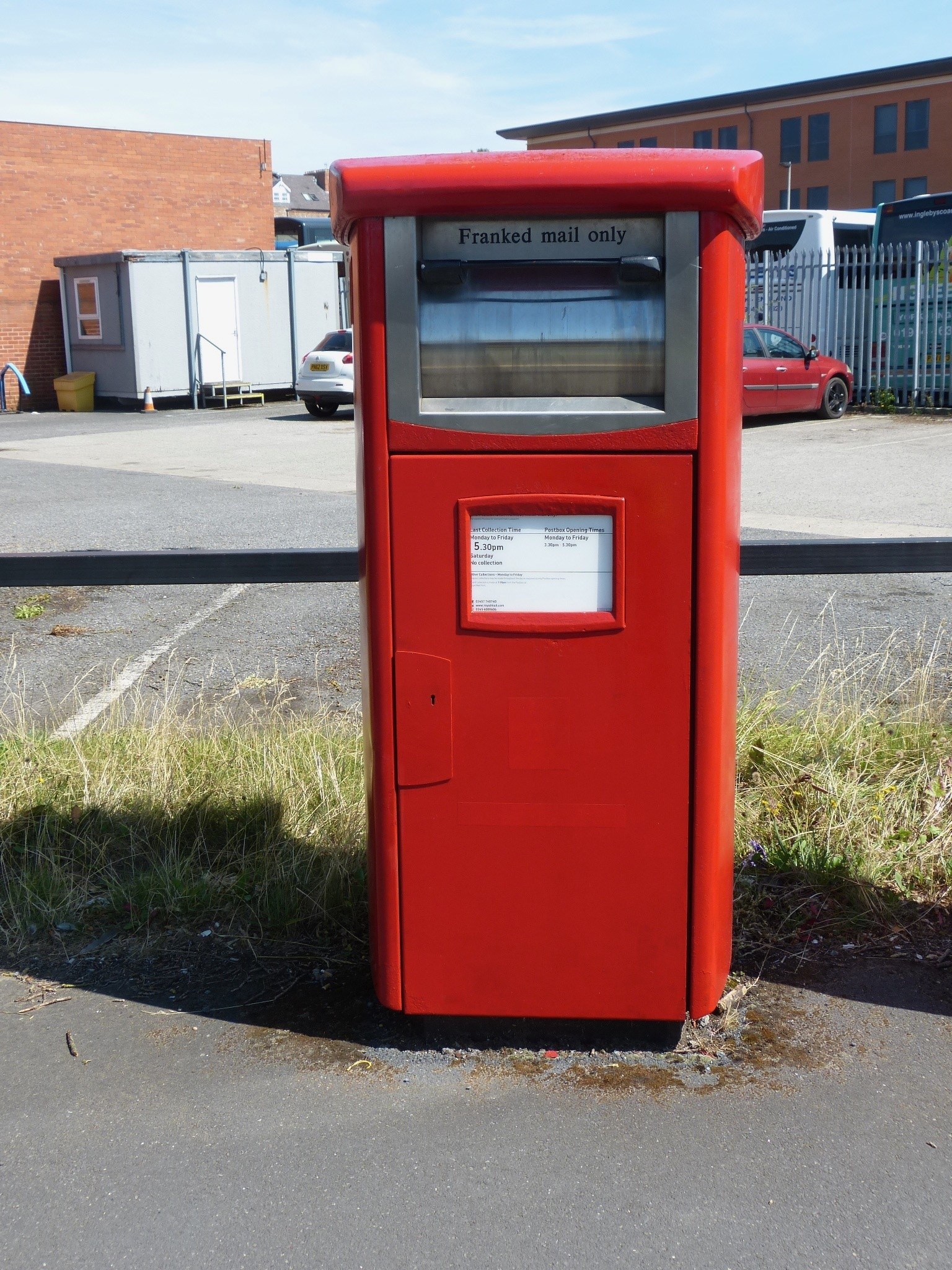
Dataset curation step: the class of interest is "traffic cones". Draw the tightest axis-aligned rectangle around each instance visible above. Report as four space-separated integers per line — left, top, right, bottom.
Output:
140 386 158 414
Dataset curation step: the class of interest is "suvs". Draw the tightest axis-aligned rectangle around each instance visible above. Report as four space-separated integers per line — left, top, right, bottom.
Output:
295 327 354 418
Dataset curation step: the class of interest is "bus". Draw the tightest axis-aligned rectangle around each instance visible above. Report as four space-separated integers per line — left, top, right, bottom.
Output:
745 207 877 388
274 216 335 250
872 190 952 392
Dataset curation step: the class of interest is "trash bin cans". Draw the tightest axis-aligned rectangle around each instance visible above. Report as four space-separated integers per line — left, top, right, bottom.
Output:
324 144 767 1041
54 370 95 412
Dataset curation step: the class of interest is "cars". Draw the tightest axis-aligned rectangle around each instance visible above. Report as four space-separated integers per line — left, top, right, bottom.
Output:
742 323 854 420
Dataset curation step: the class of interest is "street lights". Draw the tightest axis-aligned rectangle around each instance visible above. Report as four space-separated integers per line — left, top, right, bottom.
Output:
778 160 792 210
